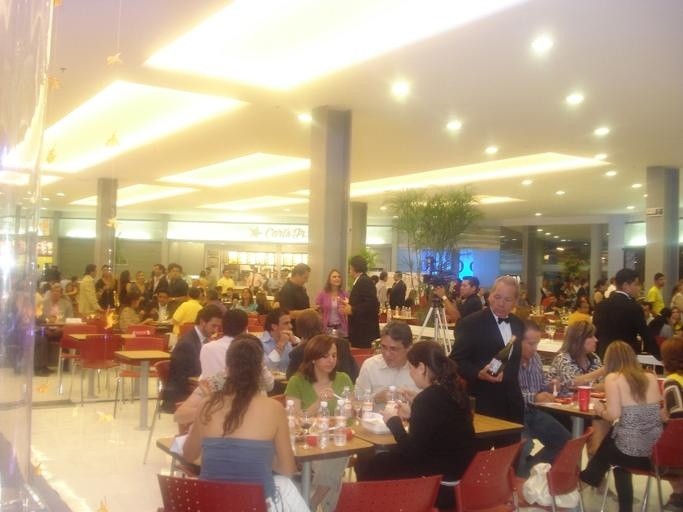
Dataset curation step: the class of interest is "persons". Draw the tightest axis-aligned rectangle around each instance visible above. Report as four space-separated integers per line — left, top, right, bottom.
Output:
182 339 480 512
434 269 682 511
5 256 428 413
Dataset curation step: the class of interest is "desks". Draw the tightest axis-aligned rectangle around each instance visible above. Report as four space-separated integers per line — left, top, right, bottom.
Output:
154 414 375 506
309 411 524 453
535 394 602 491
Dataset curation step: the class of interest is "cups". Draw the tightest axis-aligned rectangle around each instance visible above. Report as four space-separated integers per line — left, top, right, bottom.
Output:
576 384 591 412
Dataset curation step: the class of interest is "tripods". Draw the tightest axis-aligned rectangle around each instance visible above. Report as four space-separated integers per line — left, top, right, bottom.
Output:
415 295 452 357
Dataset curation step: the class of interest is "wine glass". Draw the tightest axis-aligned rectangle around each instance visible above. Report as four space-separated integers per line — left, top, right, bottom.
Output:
297 408 315 436
531 304 573 342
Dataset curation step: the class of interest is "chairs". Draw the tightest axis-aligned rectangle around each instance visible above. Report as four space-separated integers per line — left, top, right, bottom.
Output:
156 472 267 512
334 474 442 510
34 286 682 431
601 418 682 511
142 360 177 465
440 438 527 511
516 426 595 512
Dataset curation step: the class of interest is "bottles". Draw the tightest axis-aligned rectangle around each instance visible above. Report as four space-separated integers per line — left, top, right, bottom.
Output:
317 384 397 448
488 335 516 376
385 304 413 321
282 399 296 450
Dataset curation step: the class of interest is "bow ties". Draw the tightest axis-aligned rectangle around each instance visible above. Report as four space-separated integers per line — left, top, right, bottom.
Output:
498 318 510 324
